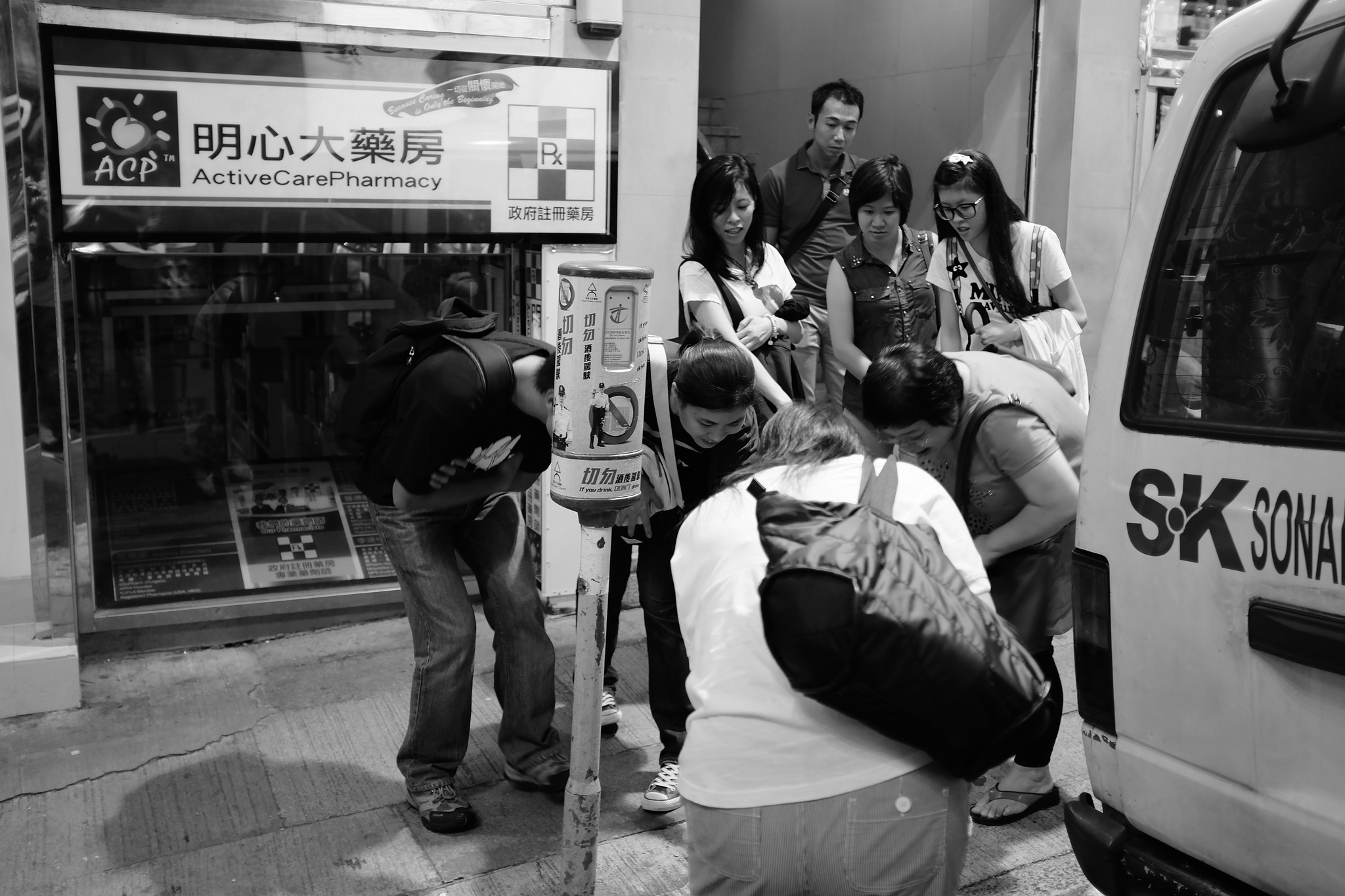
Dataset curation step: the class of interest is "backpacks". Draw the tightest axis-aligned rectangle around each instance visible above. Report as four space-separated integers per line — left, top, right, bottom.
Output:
340 295 557 461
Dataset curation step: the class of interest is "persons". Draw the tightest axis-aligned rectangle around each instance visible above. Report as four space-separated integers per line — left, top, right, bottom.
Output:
925 149 1090 415
678 153 804 439
670 399 1052 896
573 321 761 812
758 78 871 413
861 343 1088 825
316 296 570 834
826 154 942 467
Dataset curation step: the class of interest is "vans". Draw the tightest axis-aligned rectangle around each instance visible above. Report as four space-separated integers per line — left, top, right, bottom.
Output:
1056 0 1344 896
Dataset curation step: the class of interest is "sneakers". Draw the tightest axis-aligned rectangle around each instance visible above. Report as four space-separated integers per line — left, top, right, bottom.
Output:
641 762 686 811
407 785 478 833
600 690 622 726
503 755 569 794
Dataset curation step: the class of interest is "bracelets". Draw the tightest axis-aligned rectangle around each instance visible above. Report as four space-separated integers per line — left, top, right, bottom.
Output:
763 313 779 345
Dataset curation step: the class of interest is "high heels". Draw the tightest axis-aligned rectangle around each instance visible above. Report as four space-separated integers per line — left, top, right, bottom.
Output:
970 782 1060 826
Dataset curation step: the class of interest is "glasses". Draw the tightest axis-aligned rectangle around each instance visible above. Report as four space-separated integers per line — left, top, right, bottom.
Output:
933 196 984 221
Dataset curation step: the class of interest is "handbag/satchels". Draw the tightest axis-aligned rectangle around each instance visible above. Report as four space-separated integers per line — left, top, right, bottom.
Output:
985 519 1073 640
616 443 678 526
747 448 1051 779
